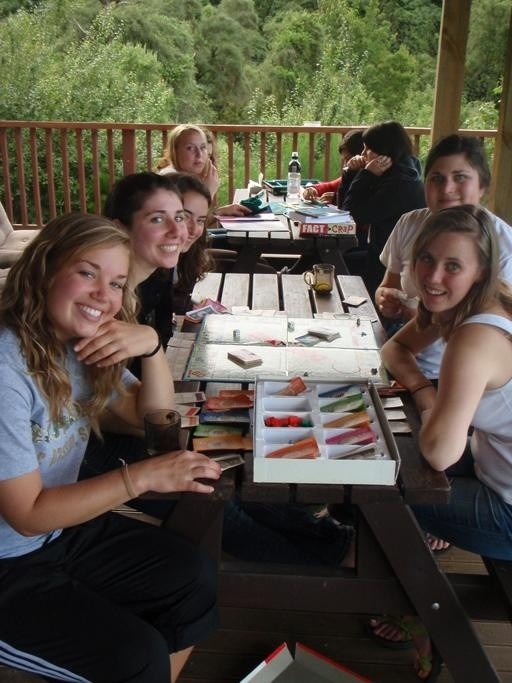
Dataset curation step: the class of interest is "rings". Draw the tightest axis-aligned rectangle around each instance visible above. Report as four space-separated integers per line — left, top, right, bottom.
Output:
377 159 383 163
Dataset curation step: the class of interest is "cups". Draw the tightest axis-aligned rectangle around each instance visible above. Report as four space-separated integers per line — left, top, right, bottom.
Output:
143 408 181 457
303 263 336 295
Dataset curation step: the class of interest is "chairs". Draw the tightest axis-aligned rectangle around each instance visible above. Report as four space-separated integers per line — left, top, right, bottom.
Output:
0 197 41 268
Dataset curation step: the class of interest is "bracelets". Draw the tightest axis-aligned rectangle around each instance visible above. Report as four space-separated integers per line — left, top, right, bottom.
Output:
409 383 435 395
142 323 164 358
120 462 139 502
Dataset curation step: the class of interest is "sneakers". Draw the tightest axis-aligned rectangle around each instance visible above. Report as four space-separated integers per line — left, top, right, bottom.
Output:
314 514 354 567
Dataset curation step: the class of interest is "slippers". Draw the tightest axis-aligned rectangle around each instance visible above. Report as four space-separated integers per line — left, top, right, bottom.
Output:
361 612 445 681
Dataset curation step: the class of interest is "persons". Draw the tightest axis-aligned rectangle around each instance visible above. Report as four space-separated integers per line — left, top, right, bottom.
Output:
276 118 426 298
372 131 512 555
0 210 226 681
301 127 370 250
358 202 512 683
163 170 217 289
98 169 375 578
201 124 242 251
153 122 278 275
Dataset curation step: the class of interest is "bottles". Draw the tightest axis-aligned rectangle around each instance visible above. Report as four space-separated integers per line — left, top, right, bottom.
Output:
286 151 302 205
